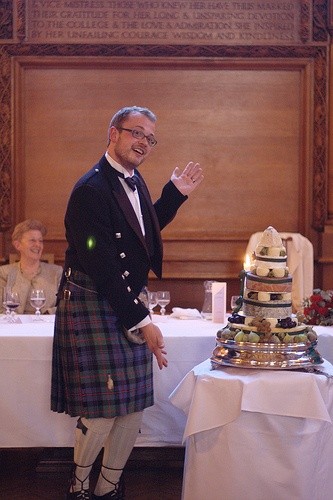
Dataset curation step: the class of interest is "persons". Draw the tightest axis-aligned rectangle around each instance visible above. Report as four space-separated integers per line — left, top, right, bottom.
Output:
0 220 63 315
50 107 206 500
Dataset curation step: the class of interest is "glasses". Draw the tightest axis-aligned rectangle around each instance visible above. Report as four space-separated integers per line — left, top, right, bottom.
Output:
114 125 158 147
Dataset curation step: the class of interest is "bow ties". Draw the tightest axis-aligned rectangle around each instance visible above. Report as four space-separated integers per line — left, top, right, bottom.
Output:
111 166 140 191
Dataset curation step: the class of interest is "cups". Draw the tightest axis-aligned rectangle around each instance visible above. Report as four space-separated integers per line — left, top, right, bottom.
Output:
231 295 243 310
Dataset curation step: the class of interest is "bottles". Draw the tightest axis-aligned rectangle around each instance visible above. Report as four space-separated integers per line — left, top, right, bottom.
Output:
200 280 216 320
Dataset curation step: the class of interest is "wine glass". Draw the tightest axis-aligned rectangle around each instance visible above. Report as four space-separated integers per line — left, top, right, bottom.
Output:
156 290 170 316
1 287 21 323
147 291 159 318
30 289 46 322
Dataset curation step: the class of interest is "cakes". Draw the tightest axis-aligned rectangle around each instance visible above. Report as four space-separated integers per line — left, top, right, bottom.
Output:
217 226 318 344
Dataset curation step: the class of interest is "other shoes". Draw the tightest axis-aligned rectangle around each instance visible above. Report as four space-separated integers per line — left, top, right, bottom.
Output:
69 477 90 500
93 480 125 500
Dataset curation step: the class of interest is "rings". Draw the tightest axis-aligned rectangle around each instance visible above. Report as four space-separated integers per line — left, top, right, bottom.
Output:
191 178 195 183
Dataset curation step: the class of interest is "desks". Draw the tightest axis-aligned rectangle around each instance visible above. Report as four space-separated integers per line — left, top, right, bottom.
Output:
169 357 333 500
0 313 333 446
